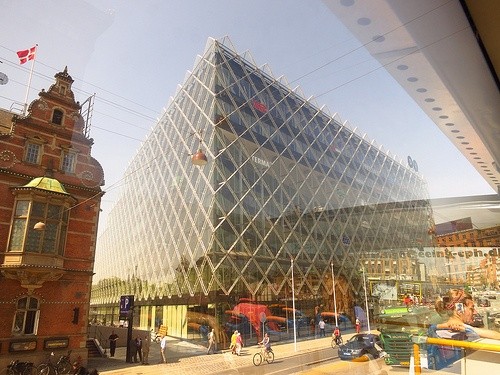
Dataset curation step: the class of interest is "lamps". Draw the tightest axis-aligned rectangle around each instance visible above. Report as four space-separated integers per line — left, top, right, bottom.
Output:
33 221 46 230
192 129 207 166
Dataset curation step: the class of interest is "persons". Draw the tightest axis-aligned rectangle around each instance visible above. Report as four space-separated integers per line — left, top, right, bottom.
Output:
89 316 129 328
159 335 167 364
108 331 119 357
319 319 326 336
134 334 142 363
258 333 270 362
141 335 151 364
356 317 361 334
207 329 218 355
297 284 500 342
334 327 341 345
229 330 245 355
68 362 87 375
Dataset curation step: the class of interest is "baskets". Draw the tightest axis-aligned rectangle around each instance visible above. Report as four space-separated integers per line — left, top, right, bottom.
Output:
331 333 336 339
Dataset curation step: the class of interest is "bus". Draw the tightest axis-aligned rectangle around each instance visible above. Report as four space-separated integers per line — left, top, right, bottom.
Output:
375 303 468 366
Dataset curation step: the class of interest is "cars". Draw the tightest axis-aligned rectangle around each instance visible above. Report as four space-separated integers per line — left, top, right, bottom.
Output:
473 293 496 300
338 333 381 360
476 298 491 307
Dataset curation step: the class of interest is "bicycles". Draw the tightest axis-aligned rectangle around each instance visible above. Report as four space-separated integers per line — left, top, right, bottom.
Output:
253 346 274 367
150 329 162 344
34 353 72 375
407 302 420 313
331 335 343 349
0 360 38 375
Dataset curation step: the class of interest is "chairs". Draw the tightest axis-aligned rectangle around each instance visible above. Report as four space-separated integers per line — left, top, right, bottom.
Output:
426 325 480 370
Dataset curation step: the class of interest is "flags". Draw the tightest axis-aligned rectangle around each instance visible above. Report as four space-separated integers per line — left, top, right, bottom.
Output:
17 47 35 65
